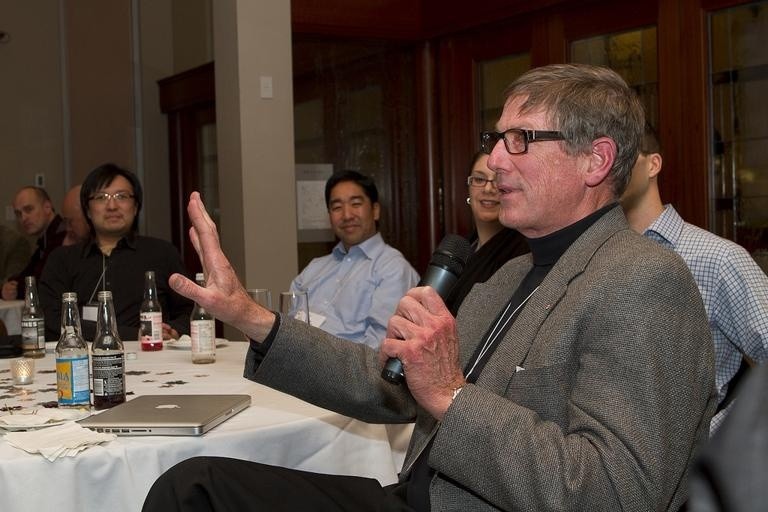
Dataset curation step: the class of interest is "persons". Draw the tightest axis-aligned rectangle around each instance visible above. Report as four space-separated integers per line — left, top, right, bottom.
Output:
431 148 529 320
617 117 767 435
1 165 192 341
139 63 716 511
289 168 421 353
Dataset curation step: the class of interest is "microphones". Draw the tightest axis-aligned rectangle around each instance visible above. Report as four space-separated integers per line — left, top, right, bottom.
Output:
381 232 475 385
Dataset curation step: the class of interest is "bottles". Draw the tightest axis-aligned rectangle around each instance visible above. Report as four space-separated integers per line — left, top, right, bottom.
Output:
93 291 126 409
140 271 163 350
21 276 45 357
190 273 215 363
56 292 91 409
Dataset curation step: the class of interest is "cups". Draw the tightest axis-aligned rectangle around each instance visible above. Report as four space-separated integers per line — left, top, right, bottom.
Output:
10 359 34 384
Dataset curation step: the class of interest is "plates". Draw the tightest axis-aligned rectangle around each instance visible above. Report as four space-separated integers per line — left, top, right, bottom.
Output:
168 338 228 347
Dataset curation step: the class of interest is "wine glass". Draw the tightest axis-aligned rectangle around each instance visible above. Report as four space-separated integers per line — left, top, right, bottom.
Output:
246 288 270 341
279 291 310 325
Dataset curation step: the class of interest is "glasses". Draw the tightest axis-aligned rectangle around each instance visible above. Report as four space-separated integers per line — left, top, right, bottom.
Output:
480 128 573 153
468 176 497 188
87 191 134 204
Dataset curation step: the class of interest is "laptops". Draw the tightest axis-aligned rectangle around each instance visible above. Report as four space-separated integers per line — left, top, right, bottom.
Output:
77 396 251 435
